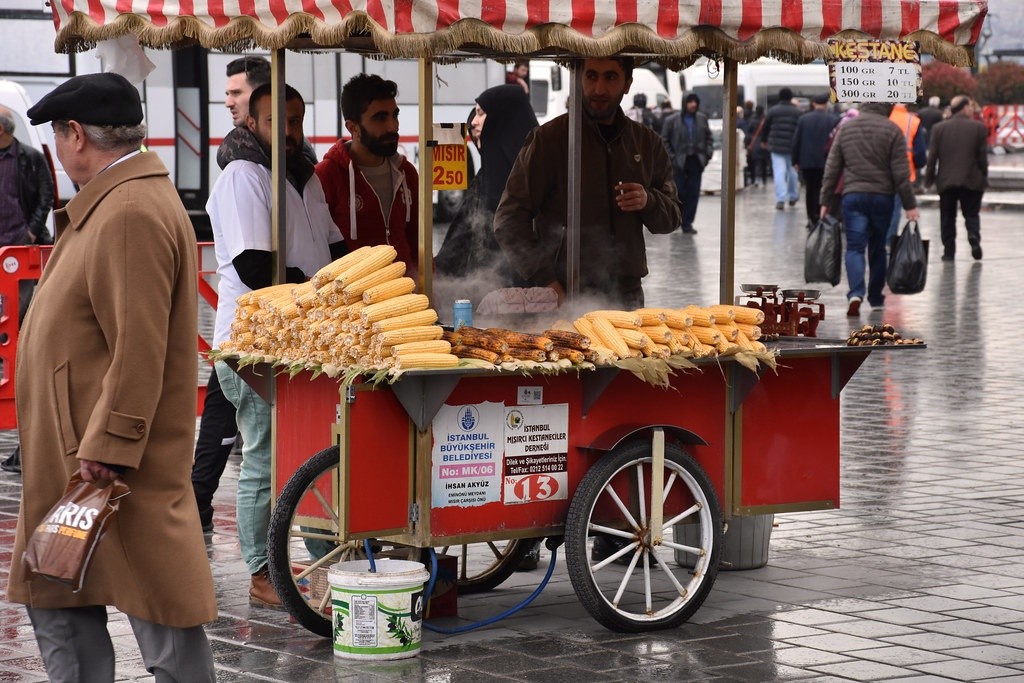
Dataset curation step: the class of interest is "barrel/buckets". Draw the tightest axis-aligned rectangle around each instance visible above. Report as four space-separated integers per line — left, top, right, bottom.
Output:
327 560 430 660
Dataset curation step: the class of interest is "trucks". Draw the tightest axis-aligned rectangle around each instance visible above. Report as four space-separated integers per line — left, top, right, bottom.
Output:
47 0 992 640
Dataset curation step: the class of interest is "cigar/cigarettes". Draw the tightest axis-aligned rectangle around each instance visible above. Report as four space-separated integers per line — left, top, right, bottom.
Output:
619 181 624 196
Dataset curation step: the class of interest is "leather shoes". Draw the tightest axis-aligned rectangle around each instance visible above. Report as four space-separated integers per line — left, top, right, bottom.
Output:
248 571 289 612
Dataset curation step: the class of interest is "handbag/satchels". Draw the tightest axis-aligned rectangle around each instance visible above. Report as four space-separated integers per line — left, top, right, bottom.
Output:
886 220 930 295
747 146 753 159
804 214 842 287
21 467 132 595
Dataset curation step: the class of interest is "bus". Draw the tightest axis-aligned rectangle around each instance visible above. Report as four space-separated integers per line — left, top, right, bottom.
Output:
0 0 831 242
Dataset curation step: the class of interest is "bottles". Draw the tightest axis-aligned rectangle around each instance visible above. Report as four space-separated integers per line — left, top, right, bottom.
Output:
453 300 473 333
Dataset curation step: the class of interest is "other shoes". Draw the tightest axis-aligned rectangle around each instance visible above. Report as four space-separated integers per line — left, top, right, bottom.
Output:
968 236 983 260
872 305 884 311
776 202 784 209
789 192 800 205
847 296 862 316
682 227 698 234
942 255 954 261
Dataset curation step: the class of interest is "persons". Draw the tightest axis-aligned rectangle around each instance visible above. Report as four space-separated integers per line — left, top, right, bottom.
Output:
0 111 55 362
205 82 366 613
5 73 219 683
313 73 436 295
191 56 318 533
624 93 675 134
662 91 712 234
820 103 919 317
735 90 990 262
494 50 684 312
433 59 542 308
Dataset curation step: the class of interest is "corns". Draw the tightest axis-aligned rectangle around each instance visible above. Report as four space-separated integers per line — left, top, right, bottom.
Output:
219 243 460 370
552 305 767 366
441 324 598 366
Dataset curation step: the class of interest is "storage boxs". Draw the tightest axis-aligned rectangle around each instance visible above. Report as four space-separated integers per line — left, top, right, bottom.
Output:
287 548 459 623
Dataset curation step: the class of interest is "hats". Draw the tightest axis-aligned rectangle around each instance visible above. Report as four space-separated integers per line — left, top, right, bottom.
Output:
26 72 144 128
